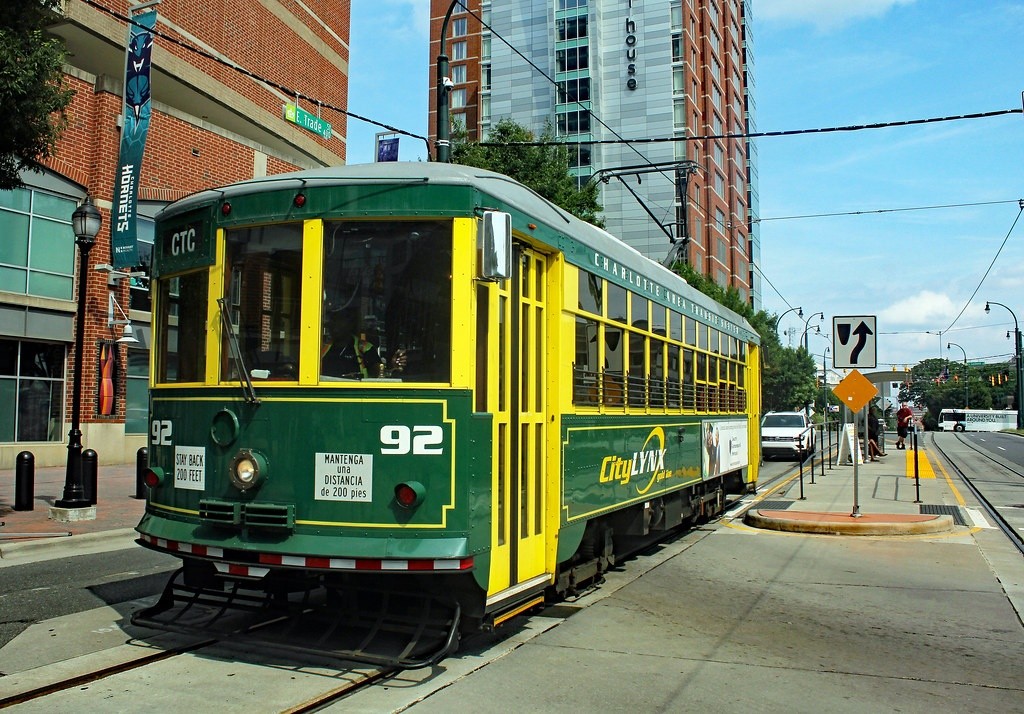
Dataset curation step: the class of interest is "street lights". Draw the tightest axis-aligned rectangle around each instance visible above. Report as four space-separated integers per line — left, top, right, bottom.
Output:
800 311 825 354
985 301 1024 429
52 193 103 509
775 307 803 334
947 342 968 409
823 347 830 431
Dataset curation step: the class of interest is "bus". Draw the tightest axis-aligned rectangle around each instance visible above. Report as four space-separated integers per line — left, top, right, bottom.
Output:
937 409 1018 432
135 162 763 671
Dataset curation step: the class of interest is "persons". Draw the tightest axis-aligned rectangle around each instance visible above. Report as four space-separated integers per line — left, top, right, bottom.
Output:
704 422 720 477
320 304 408 380
894 400 914 450
861 406 881 458
858 417 889 462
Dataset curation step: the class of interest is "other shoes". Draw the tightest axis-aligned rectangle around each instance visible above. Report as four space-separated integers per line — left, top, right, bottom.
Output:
880 452 887 457
895 441 906 449
871 458 879 461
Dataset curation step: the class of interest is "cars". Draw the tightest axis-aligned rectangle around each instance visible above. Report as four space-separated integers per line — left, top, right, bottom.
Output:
758 411 817 461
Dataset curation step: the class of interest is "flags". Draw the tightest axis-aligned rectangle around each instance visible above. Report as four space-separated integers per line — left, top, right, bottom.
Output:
377 137 399 162
111 9 158 269
